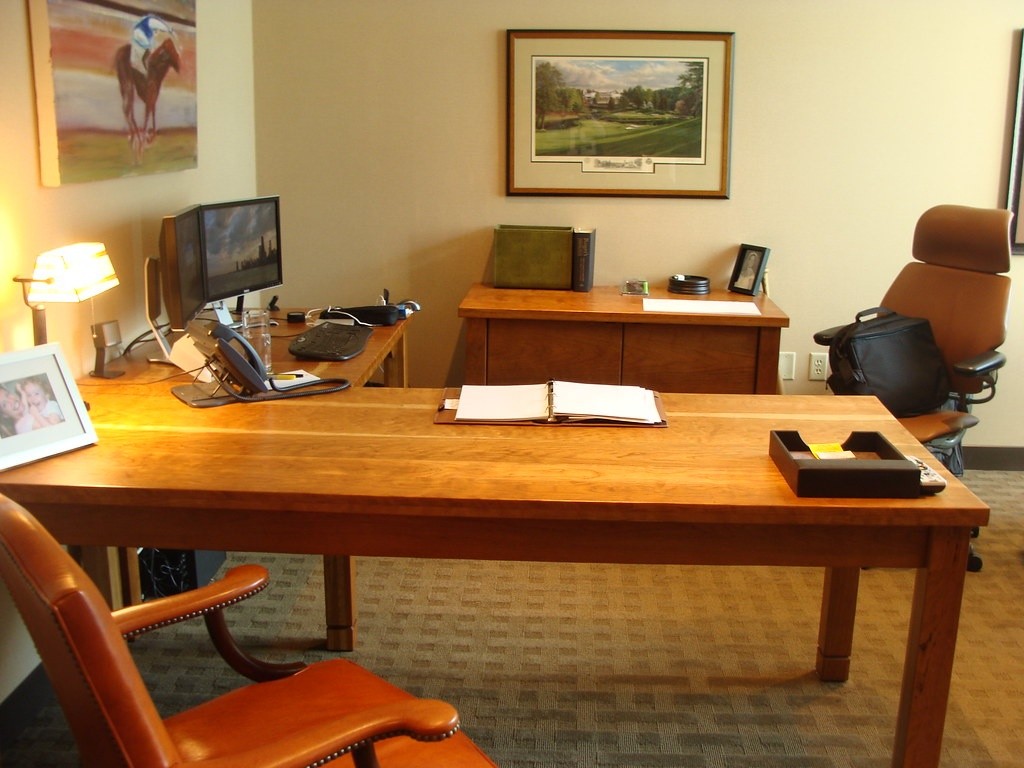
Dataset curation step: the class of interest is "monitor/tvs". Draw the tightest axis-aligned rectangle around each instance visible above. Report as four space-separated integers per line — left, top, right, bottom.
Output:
144 196 280 366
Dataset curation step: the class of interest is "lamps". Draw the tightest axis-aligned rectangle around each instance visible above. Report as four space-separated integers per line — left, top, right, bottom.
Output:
13 241 119 346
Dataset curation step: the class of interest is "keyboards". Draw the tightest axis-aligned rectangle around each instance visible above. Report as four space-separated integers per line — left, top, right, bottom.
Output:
288 321 374 361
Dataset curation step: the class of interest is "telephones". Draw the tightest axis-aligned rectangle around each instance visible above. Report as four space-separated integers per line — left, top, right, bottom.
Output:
187 319 268 397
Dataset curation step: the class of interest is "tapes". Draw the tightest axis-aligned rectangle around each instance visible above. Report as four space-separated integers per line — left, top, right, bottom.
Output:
287 312 305 322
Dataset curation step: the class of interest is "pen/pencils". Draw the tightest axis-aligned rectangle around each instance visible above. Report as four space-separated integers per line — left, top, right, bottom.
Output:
265 374 305 381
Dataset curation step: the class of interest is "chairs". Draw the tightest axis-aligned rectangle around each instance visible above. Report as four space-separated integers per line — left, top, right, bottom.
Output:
0 492 496 768
813 204 1015 572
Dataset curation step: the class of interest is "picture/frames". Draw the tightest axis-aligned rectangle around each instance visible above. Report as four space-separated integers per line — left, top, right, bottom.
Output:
727 244 771 296
0 341 100 474
506 29 733 200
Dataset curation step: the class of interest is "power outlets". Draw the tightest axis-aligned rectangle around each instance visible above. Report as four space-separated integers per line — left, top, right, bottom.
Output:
808 352 829 382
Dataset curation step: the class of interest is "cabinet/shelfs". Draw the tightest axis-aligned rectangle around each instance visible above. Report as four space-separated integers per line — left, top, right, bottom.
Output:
459 282 788 396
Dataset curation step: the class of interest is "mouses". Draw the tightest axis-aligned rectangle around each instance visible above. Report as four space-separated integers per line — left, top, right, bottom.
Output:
397 300 420 311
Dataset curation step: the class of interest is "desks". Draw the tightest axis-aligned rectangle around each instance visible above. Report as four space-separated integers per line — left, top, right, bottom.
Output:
0 308 991 768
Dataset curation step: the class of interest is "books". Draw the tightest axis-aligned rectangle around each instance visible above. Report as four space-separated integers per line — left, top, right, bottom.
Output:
574 229 596 293
434 380 668 429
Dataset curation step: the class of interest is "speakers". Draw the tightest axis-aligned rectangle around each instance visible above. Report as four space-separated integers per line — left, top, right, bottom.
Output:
89 320 126 379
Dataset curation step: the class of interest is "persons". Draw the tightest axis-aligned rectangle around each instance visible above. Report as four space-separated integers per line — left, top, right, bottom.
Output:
735 252 759 290
0 379 64 438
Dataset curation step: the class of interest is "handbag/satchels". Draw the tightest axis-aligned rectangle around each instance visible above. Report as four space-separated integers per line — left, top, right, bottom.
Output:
826 307 951 418
